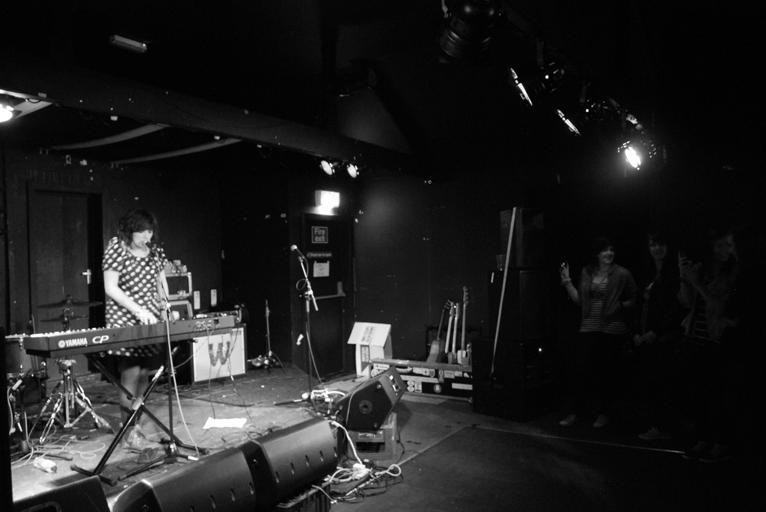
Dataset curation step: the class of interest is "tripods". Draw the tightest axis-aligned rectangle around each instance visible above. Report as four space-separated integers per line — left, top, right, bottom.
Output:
119 251 203 481
27 359 113 446
256 296 286 376
274 254 347 415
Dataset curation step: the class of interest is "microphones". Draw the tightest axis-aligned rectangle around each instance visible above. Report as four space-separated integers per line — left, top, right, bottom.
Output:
144 239 167 260
291 244 308 262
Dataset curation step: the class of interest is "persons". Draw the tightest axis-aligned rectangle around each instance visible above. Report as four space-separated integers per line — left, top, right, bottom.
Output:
670 227 746 439
632 228 688 442
99 207 175 455
555 238 639 433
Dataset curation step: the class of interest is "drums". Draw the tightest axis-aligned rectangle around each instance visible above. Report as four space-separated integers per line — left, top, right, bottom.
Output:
6 334 46 379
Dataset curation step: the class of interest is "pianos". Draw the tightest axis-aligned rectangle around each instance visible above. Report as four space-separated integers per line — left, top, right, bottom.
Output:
23 312 237 358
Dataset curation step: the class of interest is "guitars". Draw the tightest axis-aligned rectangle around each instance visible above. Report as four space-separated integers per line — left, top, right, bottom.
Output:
427 286 472 365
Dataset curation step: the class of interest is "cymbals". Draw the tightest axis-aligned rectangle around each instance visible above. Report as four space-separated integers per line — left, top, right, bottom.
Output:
36 299 103 308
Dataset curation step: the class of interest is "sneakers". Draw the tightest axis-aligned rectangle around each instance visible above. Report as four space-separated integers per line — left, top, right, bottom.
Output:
123 425 160 453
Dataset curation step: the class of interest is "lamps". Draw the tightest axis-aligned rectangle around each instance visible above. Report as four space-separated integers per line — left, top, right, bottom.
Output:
317 156 361 179
439 0 656 178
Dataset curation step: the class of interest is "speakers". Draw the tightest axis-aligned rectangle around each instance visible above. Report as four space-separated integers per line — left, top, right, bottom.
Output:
333 364 408 434
361 136 396 175
485 268 560 339
470 338 564 423
240 415 339 502
13 475 111 512
108 447 260 512
499 206 557 270
